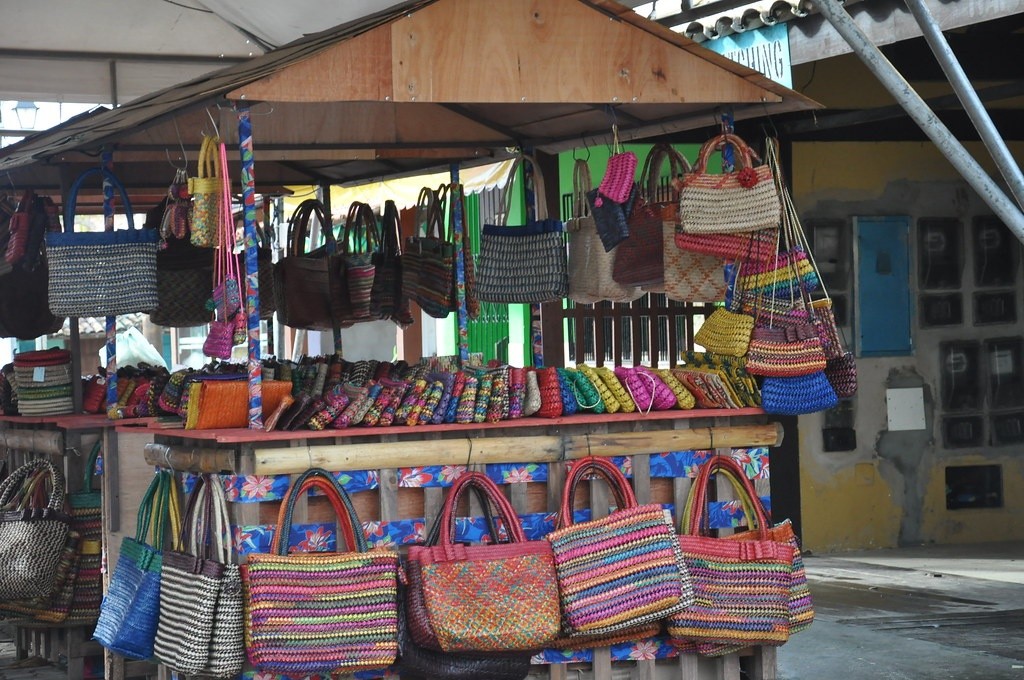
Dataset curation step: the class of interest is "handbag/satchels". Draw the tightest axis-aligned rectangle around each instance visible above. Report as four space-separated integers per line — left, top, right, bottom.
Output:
0 130 856 680
